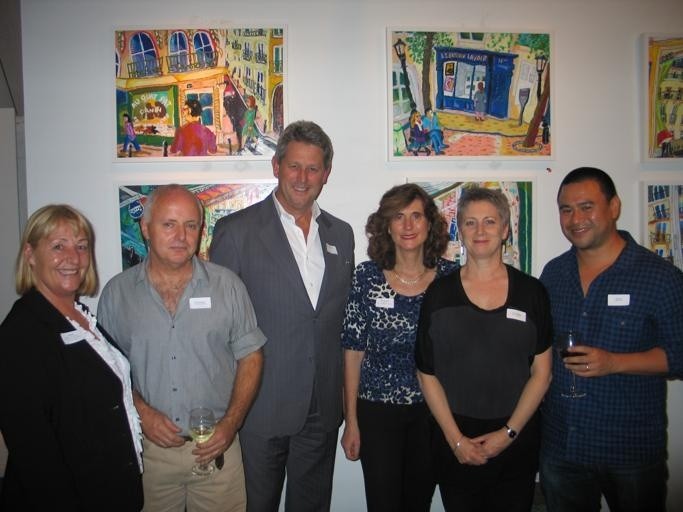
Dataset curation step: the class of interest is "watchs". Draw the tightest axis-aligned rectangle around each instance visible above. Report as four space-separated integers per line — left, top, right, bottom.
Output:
501 424 516 440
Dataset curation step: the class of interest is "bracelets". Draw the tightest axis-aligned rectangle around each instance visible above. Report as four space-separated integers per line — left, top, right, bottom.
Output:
447 433 464 458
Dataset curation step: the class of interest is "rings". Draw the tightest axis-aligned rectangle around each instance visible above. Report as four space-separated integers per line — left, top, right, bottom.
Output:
584 364 589 371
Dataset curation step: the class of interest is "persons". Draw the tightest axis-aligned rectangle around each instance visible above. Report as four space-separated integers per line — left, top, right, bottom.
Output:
413 186 555 512
208 119 357 511
535 165 682 511
96 184 269 512
0 204 144 512
341 182 461 512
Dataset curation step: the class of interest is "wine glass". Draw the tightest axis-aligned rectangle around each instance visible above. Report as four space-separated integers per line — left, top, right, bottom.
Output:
557 329 586 402
186 407 215 477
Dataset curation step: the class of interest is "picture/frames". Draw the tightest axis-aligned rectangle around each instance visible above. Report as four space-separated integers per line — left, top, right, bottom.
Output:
643 32 683 163
638 180 683 274
112 179 279 273
405 174 537 279
111 24 288 163
386 25 557 162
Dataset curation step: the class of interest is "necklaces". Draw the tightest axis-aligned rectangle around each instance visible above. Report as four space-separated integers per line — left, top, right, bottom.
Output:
392 267 430 286
